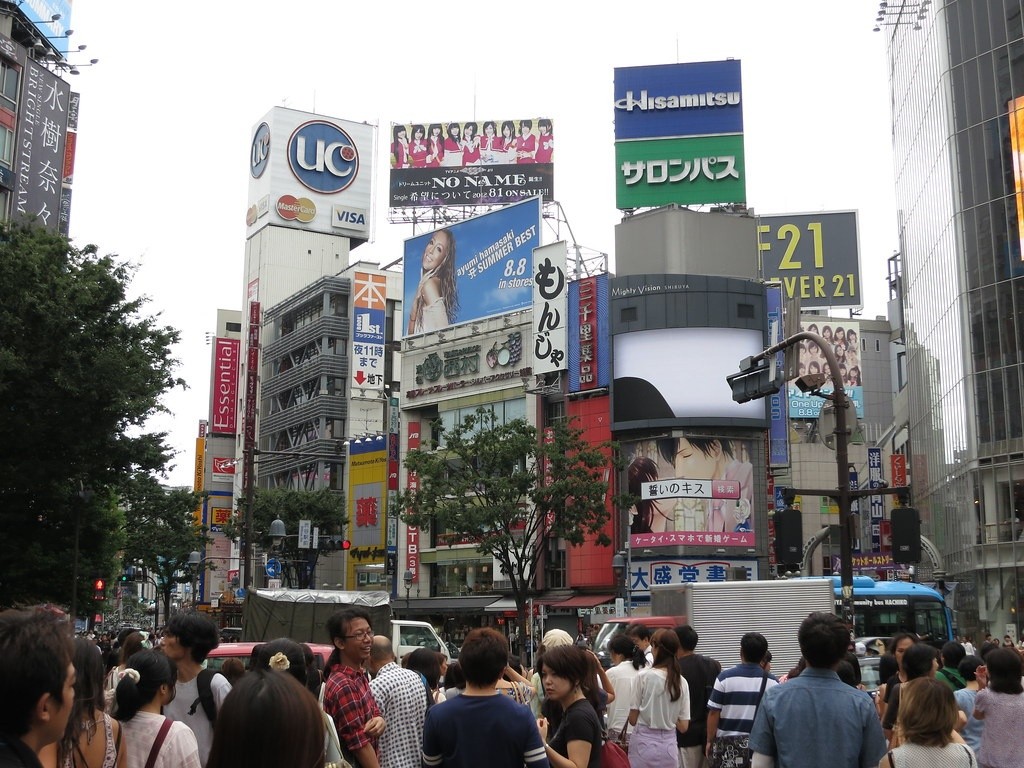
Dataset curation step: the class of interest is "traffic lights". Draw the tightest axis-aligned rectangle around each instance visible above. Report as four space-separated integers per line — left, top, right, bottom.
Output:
117 573 136 581
318 539 350 550
732 367 785 404
93 579 106 601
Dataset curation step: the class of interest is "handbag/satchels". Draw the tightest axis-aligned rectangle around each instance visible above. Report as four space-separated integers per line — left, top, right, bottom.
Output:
598 740 631 768
612 740 630 754
325 760 352 768
711 735 753 768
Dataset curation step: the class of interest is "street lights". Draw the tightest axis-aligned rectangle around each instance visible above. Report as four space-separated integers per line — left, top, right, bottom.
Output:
267 514 286 577
611 552 625 596
404 570 413 619
186 549 200 611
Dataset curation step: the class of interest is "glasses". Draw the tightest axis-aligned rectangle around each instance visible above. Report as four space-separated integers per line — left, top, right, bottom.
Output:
339 630 375 641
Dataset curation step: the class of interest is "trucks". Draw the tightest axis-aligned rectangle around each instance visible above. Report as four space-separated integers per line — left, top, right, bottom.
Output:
591 577 836 682
206 585 453 683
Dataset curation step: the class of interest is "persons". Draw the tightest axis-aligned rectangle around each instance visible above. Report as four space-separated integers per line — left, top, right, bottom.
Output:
0 600 247 768
874 633 1024 768
530 623 722 768
391 119 554 169
321 609 551 768
473 581 481 596
706 611 888 768
790 323 862 387
459 581 467 595
406 229 461 335
206 639 342 768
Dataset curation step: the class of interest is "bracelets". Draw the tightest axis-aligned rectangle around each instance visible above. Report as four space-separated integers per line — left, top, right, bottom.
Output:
410 315 416 322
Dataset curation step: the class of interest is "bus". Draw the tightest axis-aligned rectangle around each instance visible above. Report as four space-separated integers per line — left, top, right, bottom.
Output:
833 574 958 708
